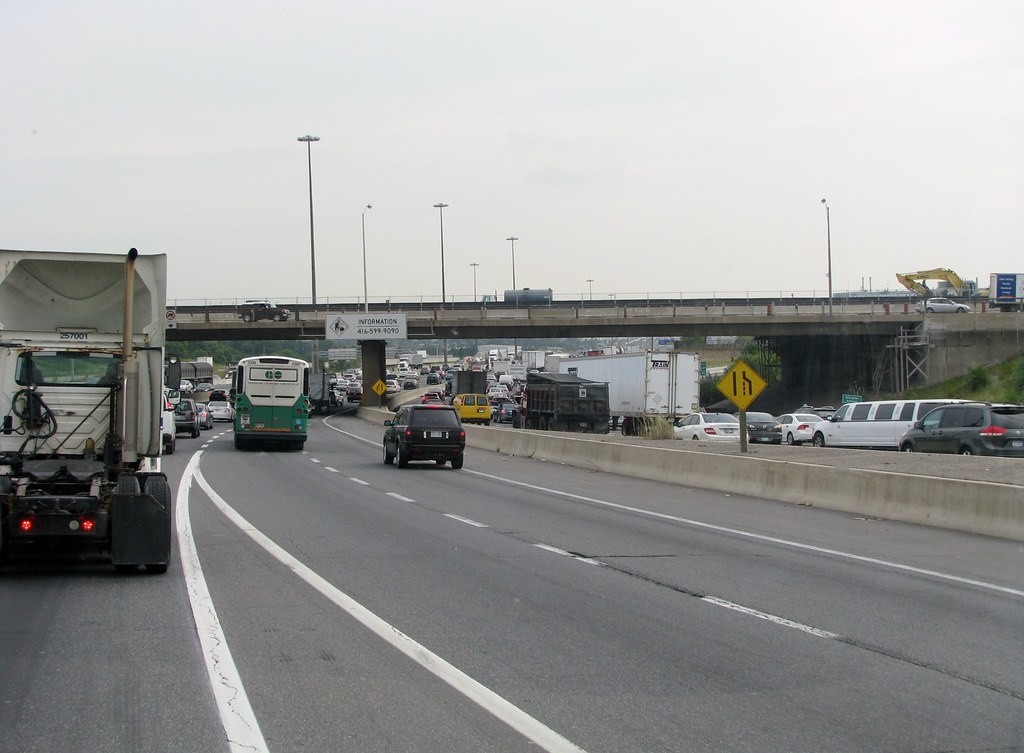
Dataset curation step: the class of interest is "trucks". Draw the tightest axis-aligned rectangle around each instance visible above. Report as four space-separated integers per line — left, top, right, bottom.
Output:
163 362 214 389
989 273 1024 313
0 248 183 580
558 350 702 436
586 344 625 357
307 372 334 414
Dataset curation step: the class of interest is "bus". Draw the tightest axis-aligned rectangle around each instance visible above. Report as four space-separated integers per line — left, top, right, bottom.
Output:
233 356 309 451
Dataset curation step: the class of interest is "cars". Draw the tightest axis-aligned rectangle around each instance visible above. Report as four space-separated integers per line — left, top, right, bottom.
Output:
161 380 237 455
915 298 971 314
775 413 825 445
733 411 782 445
811 398 972 448
673 412 749 444
328 345 569 424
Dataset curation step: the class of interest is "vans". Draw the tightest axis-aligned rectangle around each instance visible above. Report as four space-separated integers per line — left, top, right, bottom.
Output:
452 394 491 426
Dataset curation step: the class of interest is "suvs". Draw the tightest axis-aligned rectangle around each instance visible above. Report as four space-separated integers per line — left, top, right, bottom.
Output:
383 405 466 469
241 300 291 322
897 400 1024 456
792 404 836 422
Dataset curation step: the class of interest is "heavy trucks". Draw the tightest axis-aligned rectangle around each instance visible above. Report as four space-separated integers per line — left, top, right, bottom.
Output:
520 373 609 434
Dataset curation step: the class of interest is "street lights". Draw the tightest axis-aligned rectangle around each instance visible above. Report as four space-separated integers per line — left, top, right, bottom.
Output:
362 204 373 312
586 279 594 300
433 202 449 304
506 236 519 291
297 135 321 305
820 198 833 315
469 263 480 302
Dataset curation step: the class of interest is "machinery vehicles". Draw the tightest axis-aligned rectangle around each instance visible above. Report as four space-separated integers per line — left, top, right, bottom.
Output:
896 268 976 297
